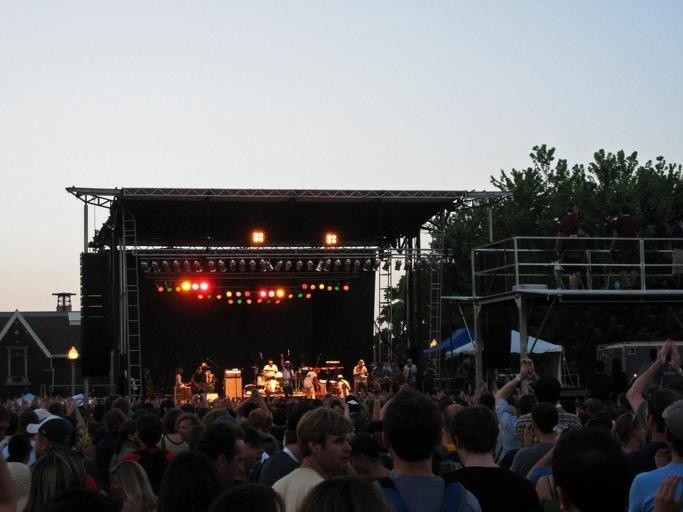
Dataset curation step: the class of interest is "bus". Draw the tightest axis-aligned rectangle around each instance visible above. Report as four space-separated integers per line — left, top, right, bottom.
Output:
596 340 682 378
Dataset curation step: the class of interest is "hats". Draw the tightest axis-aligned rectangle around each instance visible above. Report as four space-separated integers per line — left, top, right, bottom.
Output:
358 360 365 366
26 415 73 445
662 400 682 441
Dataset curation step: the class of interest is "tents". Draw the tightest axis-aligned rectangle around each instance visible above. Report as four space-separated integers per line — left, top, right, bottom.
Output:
420 328 564 388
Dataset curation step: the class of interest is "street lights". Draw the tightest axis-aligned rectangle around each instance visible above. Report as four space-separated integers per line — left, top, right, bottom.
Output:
68 346 79 397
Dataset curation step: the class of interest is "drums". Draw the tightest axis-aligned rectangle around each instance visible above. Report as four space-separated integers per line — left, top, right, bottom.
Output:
265 380 280 393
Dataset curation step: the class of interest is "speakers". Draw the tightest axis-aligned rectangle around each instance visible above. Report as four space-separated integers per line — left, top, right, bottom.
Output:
80 252 113 377
480 245 512 368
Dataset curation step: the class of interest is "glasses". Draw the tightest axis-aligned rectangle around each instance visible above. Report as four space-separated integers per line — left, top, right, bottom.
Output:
625 414 636 424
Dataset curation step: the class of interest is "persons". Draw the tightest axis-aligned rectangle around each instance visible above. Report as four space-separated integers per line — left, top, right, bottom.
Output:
671 217 683 290
0 339 682 512
554 202 581 289
610 205 640 288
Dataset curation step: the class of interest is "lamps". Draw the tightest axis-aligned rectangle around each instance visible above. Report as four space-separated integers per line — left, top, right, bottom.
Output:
138 251 458 276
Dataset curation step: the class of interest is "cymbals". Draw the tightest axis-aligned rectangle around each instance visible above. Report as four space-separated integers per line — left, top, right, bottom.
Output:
263 370 274 371
